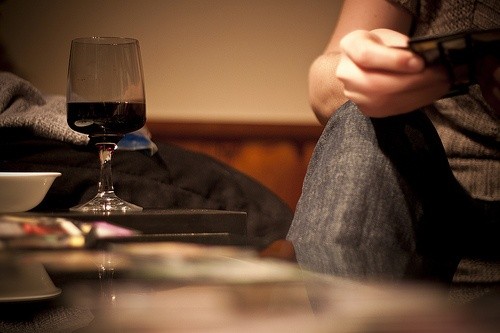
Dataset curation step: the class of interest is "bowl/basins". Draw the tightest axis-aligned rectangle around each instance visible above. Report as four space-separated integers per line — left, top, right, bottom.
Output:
1 171 62 213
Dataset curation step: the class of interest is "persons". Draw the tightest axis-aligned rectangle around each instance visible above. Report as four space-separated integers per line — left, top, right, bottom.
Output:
285 0 500 303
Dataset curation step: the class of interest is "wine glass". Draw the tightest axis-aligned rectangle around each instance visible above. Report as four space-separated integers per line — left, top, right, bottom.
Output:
66 36 146 212
